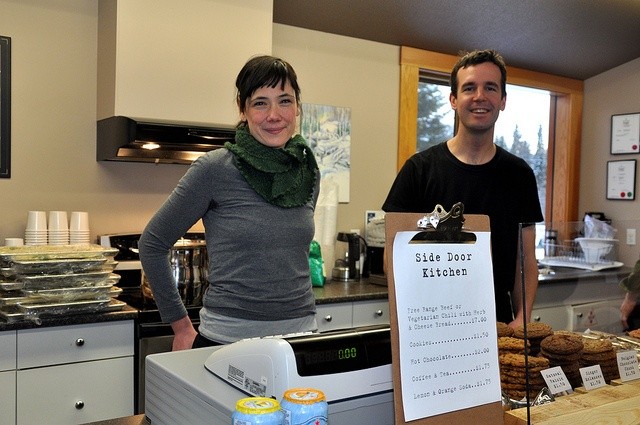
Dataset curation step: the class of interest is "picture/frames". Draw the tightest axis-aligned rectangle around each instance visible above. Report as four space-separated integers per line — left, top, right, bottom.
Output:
605 159 637 201
610 113 640 155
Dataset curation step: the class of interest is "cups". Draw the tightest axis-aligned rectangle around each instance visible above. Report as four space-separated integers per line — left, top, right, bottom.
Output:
24 211 91 245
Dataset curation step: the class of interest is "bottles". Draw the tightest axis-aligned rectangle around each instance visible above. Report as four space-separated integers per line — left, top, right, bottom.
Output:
544 229 559 258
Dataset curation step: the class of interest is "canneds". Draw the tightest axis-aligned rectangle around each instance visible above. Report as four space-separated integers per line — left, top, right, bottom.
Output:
232 398 283 425
282 389 327 425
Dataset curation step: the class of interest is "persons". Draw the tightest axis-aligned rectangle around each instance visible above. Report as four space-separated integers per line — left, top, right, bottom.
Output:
616 255 639 333
136 55 323 351
380 47 544 331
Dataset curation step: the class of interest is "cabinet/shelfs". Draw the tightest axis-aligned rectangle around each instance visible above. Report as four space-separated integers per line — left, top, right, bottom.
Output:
317 302 391 333
1 321 135 424
531 279 628 336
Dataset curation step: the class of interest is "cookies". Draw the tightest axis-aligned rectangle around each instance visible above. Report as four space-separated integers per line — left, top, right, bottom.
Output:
496 321 618 402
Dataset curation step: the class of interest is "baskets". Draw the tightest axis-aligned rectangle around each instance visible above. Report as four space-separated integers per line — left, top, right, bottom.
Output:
545 241 620 268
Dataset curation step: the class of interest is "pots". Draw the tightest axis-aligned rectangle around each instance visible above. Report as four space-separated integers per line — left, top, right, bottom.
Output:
139 238 209 307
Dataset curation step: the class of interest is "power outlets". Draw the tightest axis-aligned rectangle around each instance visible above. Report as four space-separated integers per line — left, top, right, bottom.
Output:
626 229 636 246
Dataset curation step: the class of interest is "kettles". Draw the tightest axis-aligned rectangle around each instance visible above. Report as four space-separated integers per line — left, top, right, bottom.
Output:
331 231 361 282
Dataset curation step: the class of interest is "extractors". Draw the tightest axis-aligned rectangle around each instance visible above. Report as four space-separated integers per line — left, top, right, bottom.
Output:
95 116 236 166
